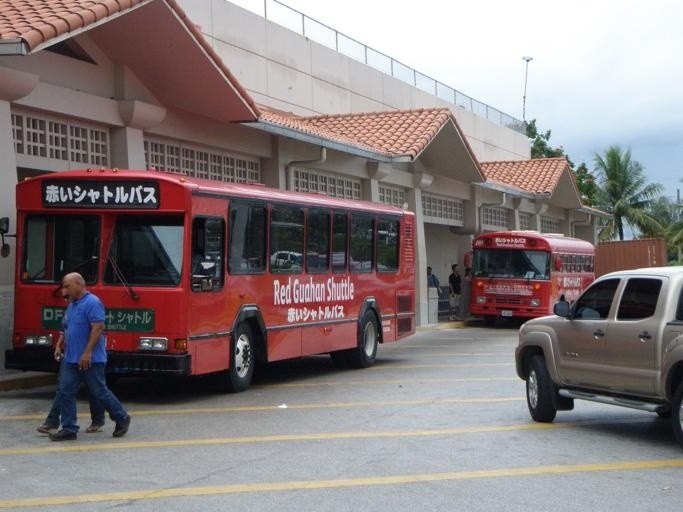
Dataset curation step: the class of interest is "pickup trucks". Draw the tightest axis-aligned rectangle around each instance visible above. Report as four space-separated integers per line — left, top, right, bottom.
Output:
514 265 682 432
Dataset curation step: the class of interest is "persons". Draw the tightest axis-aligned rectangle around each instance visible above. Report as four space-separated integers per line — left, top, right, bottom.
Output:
37 272 130 442
462 266 473 321
448 264 462 321
427 266 443 294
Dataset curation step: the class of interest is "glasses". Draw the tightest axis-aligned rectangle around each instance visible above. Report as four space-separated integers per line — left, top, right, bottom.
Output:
64 295 70 300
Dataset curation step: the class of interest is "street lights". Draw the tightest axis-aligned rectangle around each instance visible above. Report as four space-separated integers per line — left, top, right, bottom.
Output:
522 56 533 119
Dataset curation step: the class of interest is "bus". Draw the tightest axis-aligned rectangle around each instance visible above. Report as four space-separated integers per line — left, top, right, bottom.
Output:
469 229 596 320
0 168 420 392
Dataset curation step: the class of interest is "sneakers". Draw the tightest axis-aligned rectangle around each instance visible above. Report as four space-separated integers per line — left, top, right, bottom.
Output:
37 425 58 433
113 415 130 437
52 430 77 441
87 425 103 433
